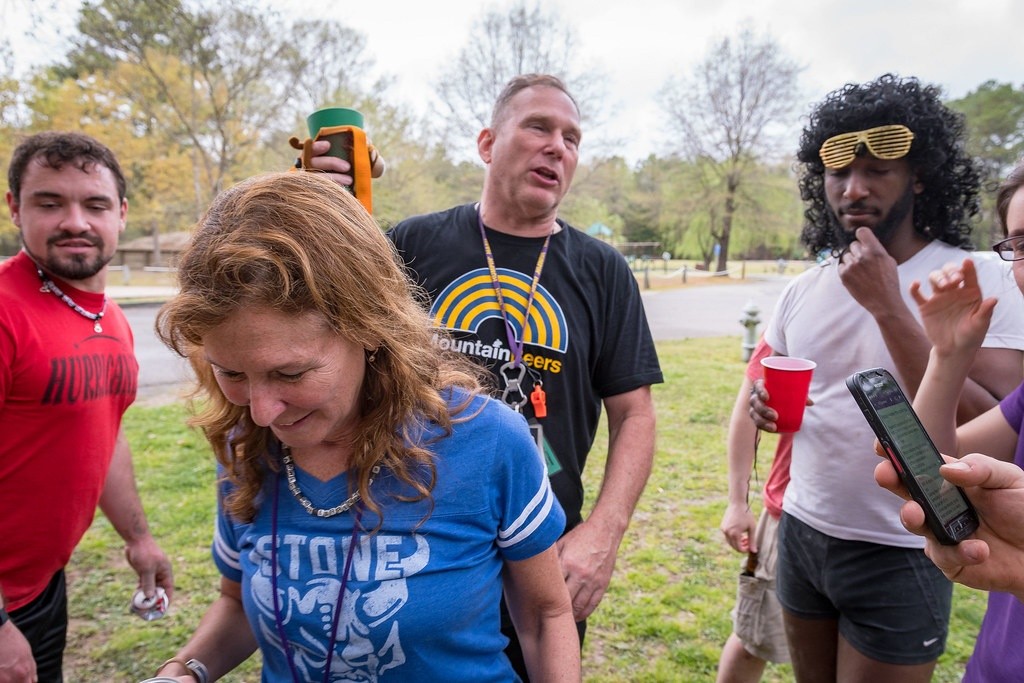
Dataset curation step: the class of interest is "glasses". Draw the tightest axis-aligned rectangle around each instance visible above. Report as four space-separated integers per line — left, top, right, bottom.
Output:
992 235 1024 261
819 125 916 170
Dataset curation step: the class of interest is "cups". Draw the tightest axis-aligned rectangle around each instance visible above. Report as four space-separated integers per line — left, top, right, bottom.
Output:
305 107 371 215
760 355 817 433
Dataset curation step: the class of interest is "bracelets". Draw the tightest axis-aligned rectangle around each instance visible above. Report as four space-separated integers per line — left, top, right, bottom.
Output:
0 608 9 627
141 656 210 683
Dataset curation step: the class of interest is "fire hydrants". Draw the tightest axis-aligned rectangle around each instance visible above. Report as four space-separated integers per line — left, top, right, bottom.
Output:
740 305 761 361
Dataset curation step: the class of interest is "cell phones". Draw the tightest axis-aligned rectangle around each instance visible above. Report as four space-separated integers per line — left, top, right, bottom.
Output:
846 367 980 545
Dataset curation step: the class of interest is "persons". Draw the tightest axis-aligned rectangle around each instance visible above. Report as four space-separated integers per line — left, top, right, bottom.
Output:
302 73 665 683
0 130 173 683
150 171 584 683
714 73 1024 683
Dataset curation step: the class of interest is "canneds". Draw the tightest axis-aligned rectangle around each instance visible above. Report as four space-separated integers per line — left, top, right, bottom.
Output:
129 586 170 621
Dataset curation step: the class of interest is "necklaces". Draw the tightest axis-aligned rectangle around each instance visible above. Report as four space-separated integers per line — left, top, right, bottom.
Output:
280 441 384 517
36 267 108 320
271 445 372 683
